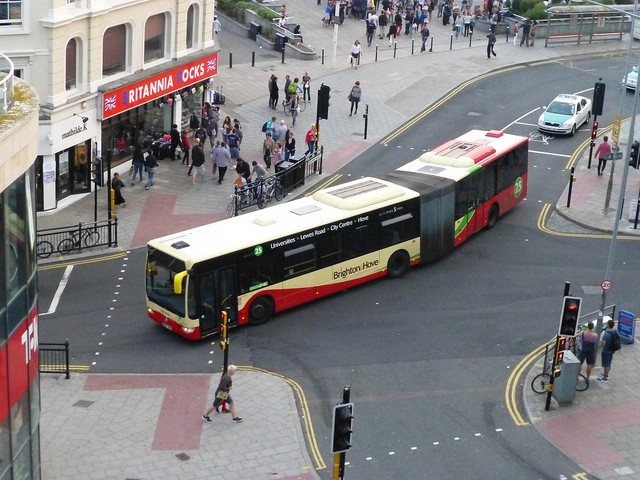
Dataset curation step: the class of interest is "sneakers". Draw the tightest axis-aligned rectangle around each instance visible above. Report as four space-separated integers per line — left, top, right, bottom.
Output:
202 415 212 423
140 179 144 183
132 181 134 185
597 378 608 383
233 417 243 422
202 179 208 183
146 187 149 190
192 183 198 187
151 182 154 186
601 373 608 378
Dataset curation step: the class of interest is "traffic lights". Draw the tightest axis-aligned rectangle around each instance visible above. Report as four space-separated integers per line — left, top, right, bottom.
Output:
629 140 640 169
320 83 331 119
557 338 565 361
219 311 227 351
90 157 104 187
558 296 582 337
592 122 598 140
331 402 354 454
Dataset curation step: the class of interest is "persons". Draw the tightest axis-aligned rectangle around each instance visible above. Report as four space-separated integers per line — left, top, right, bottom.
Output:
600 320 618 383
227 129 240 160
349 39 361 70
323 1 333 29
294 25 303 43
511 16 537 50
486 29 497 61
144 149 160 191
170 124 183 161
594 136 611 176
111 173 127 207
261 116 296 177
491 8 500 32
230 119 241 134
213 14 221 46
220 116 233 146
364 0 434 52
305 124 315 158
217 141 233 185
181 129 190 167
578 321 598 382
435 0 484 39
201 364 244 423
131 142 143 184
247 161 269 186
349 81 361 119
236 157 253 196
268 71 301 113
303 70 311 104
279 3 286 26
234 124 243 149
190 102 220 184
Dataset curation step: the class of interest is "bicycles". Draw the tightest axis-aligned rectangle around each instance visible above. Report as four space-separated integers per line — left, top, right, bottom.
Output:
226 176 284 219
37 234 52 259
284 92 306 116
58 225 99 253
531 365 589 393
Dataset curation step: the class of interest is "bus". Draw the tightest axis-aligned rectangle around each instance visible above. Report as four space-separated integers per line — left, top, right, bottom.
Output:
143 129 529 343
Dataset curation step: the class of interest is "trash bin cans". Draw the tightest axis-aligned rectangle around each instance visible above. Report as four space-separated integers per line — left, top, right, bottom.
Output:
249 21 262 41
552 349 581 406
274 33 286 51
275 154 306 192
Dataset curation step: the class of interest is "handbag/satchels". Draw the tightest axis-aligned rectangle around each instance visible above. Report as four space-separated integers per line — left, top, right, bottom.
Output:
216 377 232 399
175 144 182 155
581 332 593 353
129 165 134 175
235 176 246 188
146 159 157 173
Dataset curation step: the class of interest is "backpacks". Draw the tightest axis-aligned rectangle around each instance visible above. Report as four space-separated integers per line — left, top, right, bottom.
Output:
288 81 296 93
606 330 620 351
262 121 272 132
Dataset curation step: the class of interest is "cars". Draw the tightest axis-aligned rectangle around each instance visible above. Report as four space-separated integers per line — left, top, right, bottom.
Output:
622 65 640 90
537 94 593 137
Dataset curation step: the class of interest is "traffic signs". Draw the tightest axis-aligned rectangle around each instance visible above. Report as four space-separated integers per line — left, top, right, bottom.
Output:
600 280 612 291
